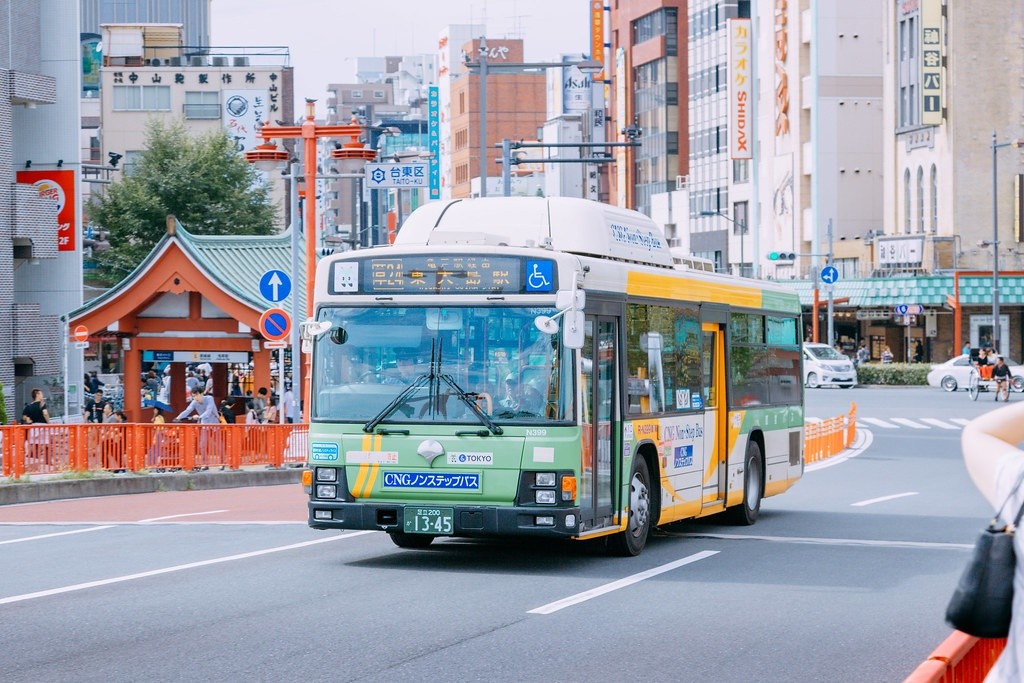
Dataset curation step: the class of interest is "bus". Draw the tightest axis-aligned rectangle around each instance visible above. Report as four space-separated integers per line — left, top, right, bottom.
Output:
302 198 805 557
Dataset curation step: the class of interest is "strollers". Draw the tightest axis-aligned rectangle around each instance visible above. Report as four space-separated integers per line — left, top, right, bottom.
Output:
156 417 201 473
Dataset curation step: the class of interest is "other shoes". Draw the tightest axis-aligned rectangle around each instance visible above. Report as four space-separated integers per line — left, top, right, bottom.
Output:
995 395 998 402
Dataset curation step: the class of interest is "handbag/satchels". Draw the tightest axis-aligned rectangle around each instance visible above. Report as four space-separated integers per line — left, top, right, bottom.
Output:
943 473 1024 639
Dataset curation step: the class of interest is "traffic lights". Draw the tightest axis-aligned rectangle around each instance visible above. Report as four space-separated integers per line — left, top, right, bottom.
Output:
767 252 796 260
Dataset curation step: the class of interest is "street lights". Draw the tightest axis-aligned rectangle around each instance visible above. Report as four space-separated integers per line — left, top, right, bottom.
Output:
464 35 603 197
977 131 1024 351
699 210 745 277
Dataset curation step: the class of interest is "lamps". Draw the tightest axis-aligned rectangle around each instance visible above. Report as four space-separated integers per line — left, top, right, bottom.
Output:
28 258 40 266
23 102 36 109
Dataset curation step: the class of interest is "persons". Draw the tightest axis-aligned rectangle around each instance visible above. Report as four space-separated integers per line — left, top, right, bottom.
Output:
962 342 970 355
526 378 546 416
835 343 845 355
980 332 992 347
805 337 810 342
991 357 1012 401
381 348 429 388
912 340 924 363
245 357 292 464
881 347 894 364
857 337 870 365
141 362 241 468
978 348 997 365
500 371 529 410
961 400 1024 683
174 387 224 470
84 371 128 473
22 388 53 465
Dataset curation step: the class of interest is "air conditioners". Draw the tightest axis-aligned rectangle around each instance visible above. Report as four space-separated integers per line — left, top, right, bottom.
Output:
213 57 229 66
151 57 166 67
233 57 249 66
190 56 207 66
169 56 187 67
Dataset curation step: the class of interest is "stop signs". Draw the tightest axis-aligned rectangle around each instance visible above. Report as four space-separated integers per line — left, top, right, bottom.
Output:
74 325 89 343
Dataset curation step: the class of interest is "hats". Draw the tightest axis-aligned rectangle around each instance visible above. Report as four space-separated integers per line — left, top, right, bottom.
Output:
506 373 518 384
394 347 421 359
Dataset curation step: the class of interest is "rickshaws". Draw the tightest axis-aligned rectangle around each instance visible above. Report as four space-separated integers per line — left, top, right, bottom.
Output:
969 348 1014 401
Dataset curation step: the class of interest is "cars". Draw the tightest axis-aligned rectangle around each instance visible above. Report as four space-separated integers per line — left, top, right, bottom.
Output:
802 341 858 389
927 351 1024 392
